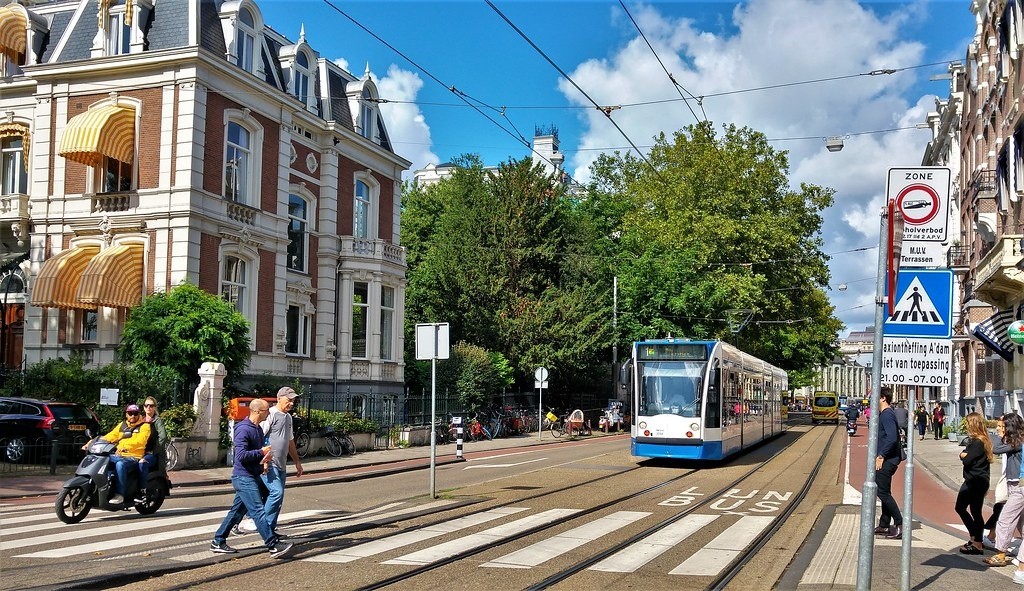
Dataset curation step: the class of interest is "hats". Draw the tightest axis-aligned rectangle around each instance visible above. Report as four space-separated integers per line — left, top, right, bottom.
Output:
278 387 299 400
127 405 139 412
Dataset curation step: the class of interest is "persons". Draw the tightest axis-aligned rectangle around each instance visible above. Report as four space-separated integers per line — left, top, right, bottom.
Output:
915 406 929 440
871 387 903 540
89 396 168 508
864 405 870 422
955 412 994 555
932 401 946 440
210 399 294 559
844 404 859 430
982 413 1024 584
229 387 303 540
893 402 908 445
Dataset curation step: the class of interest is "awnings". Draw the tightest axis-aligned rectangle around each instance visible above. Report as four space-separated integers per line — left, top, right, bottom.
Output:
76 245 143 307
0 7 26 57
31 246 100 311
58 106 135 168
973 309 1013 363
0 124 30 173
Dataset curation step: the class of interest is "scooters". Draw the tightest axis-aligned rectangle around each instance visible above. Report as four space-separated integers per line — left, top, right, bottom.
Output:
54 429 174 524
843 409 857 437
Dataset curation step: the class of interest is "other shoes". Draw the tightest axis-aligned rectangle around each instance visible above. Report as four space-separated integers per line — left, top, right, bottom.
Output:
1012 570 1024 584
983 535 996 547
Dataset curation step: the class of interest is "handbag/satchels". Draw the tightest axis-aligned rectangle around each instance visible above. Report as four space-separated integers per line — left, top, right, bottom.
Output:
900 445 907 461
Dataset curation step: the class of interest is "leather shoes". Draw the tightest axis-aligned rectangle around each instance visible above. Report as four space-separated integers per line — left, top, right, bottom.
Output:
874 526 890 535
887 525 903 539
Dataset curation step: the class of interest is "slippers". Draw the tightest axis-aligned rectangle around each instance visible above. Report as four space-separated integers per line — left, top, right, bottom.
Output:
964 540 973 546
960 543 984 554
983 554 1007 566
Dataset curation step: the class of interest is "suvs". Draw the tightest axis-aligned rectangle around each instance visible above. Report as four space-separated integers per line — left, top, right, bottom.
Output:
0 397 105 464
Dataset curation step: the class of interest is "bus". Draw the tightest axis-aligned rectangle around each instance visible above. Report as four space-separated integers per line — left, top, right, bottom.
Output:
620 309 789 460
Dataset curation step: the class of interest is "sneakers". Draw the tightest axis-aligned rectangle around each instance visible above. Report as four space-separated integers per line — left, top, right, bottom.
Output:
231 524 245 535
134 493 146 502
268 539 294 558
210 540 237 553
109 495 124 504
273 532 287 540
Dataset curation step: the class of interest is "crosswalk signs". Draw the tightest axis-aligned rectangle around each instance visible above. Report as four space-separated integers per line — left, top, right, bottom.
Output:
883 268 955 339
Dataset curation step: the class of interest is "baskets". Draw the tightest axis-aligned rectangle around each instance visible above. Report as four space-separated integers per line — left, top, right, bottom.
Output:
547 412 557 422
321 425 334 435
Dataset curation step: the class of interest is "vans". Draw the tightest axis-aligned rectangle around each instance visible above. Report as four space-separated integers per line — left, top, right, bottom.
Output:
810 390 841 425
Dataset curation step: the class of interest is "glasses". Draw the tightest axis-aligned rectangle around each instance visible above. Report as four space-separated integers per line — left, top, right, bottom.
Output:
144 404 155 408
254 408 270 412
127 411 139 416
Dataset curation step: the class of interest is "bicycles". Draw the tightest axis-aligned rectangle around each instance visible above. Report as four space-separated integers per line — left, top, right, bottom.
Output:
435 405 561 445
164 440 179 472
550 411 579 439
319 427 356 456
287 426 311 459
